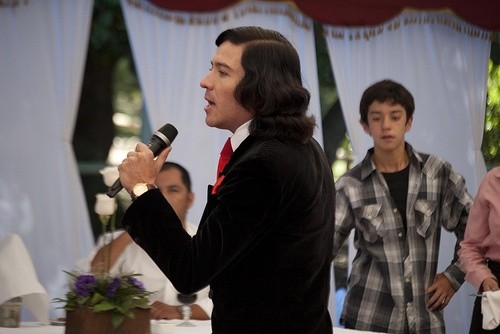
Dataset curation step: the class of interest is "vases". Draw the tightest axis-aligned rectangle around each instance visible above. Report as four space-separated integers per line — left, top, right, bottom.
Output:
65 308 151 334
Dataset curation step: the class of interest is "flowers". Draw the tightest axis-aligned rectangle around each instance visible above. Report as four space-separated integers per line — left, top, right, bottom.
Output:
50 260 160 327
94 165 120 276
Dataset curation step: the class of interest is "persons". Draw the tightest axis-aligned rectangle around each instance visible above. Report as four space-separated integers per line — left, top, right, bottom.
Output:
458 167 500 334
334 79 473 334
83 162 213 321
106 26 337 334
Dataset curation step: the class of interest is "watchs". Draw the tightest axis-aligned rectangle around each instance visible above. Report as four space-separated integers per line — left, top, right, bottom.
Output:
181 305 192 321
131 183 157 201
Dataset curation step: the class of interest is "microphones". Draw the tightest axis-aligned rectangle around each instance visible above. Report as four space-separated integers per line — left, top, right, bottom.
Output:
107 123 179 198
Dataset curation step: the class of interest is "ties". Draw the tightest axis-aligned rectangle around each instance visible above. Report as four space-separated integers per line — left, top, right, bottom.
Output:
211 136 234 195
